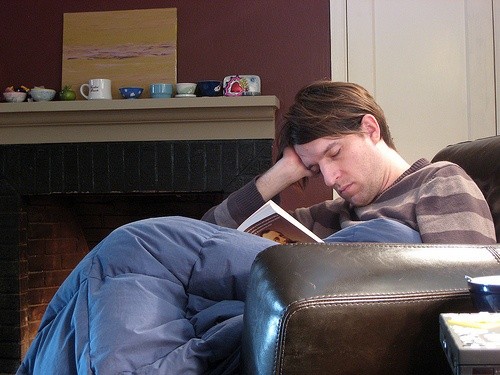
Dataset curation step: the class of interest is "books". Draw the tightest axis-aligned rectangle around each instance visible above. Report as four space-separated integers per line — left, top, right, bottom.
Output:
236 199 325 244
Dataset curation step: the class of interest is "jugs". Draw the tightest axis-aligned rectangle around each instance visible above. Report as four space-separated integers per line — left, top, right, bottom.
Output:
60 86 76 100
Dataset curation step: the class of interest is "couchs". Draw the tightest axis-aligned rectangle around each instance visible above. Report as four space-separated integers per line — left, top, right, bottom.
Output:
242 134 500 375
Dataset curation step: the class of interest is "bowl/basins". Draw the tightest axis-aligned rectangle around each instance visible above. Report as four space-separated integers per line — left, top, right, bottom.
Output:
467 275 500 313
30 88 56 102
174 83 197 95
198 80 223 96
119 87 144 99
3 92 28 102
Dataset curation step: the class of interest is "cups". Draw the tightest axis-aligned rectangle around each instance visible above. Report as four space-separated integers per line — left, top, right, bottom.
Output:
80 78 112 100
151 84 173 98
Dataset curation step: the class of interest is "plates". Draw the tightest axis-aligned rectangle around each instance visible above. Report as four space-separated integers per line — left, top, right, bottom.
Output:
175 94 196 98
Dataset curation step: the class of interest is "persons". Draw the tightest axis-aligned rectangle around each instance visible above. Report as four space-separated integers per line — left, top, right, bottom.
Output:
200 80 497 244
